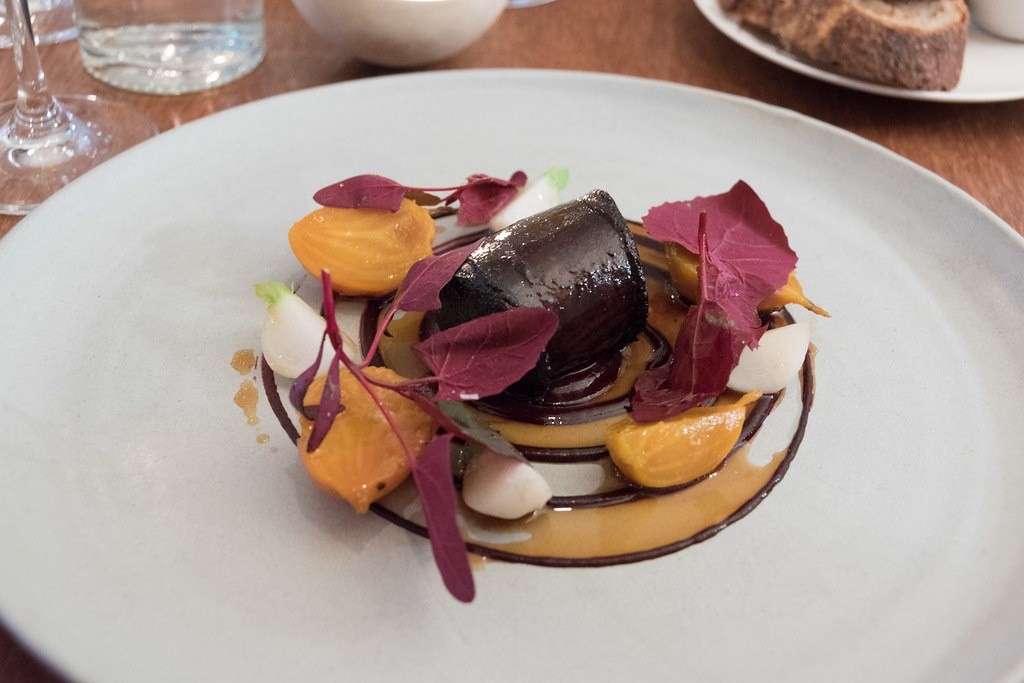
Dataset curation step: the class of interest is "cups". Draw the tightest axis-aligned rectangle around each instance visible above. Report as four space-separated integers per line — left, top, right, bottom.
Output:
968 0 1024 43
72 0 265 96
0 0 82 50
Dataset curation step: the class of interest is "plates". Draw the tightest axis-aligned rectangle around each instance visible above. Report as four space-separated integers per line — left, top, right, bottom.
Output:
0 68 1024 683
694 0 1024 103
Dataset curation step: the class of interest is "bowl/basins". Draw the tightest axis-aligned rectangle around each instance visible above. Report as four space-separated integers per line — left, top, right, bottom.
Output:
292 0 510 67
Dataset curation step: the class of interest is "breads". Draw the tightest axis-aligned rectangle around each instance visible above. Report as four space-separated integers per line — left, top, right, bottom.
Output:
717 0 972 90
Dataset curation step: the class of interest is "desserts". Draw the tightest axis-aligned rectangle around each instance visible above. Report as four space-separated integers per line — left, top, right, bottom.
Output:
437 190 650 392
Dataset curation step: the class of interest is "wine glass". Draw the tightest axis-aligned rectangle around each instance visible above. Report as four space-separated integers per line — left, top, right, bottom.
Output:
0 0 161 217
